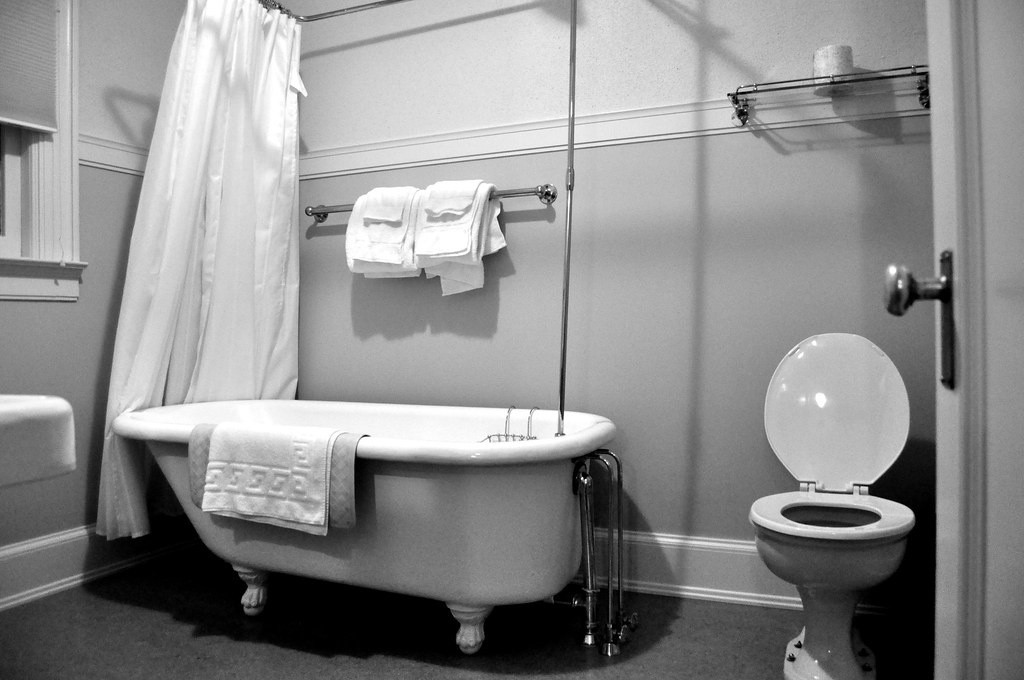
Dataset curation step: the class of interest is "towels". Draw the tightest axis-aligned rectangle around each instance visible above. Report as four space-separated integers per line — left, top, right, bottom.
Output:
344 178 508 297
186 421 372 536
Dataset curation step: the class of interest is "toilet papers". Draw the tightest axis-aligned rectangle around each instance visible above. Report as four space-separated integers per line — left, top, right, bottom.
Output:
813 43 853 99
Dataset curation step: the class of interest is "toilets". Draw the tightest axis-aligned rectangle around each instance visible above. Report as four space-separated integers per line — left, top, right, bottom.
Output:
746 331 917 679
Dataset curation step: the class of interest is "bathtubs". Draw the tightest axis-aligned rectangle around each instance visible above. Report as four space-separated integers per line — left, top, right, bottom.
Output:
110 399 618 653
